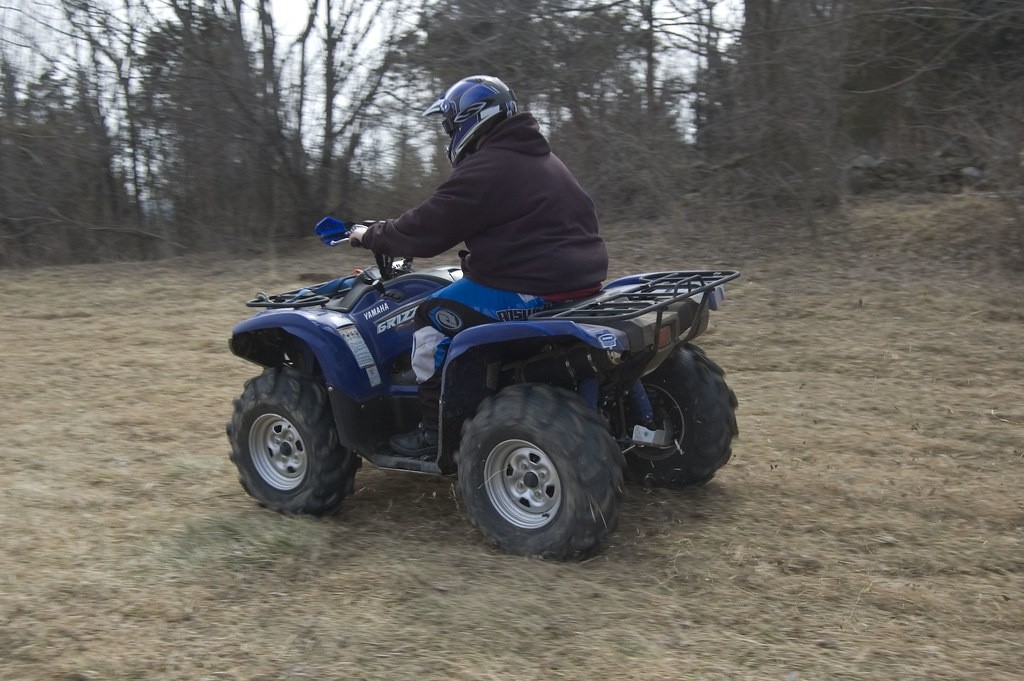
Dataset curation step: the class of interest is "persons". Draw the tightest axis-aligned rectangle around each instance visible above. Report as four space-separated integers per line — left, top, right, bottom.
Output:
346 74 609 456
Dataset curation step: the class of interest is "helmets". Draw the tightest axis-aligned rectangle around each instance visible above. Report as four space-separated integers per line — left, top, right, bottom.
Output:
422 76 517 169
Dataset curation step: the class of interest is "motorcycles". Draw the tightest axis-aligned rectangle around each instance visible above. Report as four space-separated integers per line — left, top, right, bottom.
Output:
225 214 743 561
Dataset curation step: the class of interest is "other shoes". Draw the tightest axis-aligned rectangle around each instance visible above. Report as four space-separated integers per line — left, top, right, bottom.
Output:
389 424 440 455
